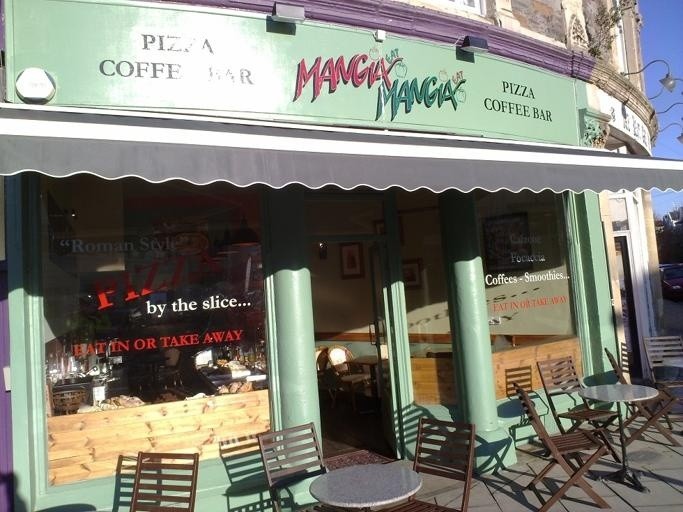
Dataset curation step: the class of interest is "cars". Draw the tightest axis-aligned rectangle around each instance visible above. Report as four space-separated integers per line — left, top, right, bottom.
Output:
657 262 682 303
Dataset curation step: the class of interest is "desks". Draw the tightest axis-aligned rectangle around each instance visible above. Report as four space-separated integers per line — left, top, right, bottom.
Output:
578 384 659 421
343 354 384 416
54 375 128 404
309 463 424 511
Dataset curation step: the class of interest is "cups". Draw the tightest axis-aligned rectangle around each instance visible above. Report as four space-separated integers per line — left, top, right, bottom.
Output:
47 341 115 383
207 339 268 378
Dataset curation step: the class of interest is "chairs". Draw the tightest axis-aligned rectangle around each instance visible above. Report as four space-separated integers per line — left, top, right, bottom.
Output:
326 345 371 411
380 416 476 512
316 347 334 401
537 356 622 475
52 390 88 416
643 336 683 430
258 421 328 512
513 383 612 512
130 451 199 511
603 347 681 448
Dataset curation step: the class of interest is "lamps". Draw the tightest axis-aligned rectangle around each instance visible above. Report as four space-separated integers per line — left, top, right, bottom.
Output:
217 229 240 254
210 236 227 261
622 59 683 146
271 1 305 24
450 35 488 54
229 214 260 247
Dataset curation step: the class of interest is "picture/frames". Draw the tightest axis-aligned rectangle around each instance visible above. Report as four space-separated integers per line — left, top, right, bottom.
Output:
402 257 425 292
481 211 534 273
339 242 364 279
